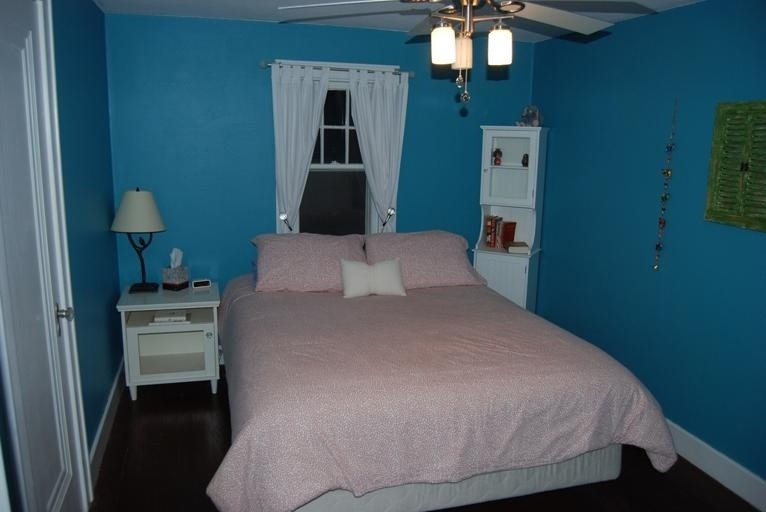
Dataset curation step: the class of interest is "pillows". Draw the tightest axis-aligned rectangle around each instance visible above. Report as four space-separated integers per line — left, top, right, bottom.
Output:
366 231 487 287
249 233 366 294
338 256 408 298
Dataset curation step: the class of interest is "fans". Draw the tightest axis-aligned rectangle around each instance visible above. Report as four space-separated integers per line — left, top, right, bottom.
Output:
274 0 616 38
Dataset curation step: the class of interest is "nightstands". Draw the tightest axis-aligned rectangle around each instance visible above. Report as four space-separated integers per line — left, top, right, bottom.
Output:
115 280 221 401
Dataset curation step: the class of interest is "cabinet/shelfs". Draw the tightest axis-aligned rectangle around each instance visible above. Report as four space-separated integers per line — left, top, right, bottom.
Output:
472 124 551 314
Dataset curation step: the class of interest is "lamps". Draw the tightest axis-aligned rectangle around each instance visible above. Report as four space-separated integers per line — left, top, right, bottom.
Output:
109 184 166 293
405 0 525 102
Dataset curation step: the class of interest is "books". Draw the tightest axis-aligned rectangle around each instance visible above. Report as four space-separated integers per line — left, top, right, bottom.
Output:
149 313 191 325
486 215 528 253
153 310 186 322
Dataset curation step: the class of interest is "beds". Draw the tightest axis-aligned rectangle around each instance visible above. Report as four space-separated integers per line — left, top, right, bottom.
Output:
206 269 679 512
